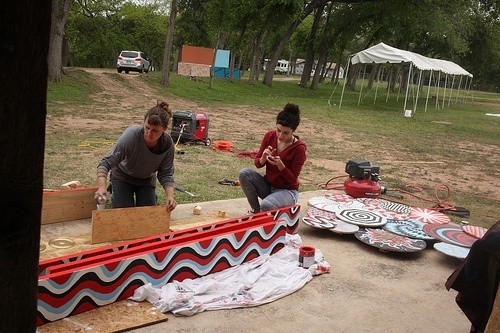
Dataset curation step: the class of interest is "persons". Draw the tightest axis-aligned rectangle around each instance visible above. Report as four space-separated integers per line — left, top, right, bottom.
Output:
94 102 178 213
239 103 307 215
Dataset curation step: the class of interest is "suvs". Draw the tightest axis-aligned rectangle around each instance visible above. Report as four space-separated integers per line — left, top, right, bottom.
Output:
117 51 150 73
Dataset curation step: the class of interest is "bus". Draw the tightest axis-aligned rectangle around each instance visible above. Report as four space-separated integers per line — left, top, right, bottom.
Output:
264 59 291 75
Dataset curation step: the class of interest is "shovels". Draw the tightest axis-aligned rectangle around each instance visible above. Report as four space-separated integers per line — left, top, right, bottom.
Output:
175 187 201 197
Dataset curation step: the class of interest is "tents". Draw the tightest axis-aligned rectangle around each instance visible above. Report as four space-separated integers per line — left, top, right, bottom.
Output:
327 41 475 114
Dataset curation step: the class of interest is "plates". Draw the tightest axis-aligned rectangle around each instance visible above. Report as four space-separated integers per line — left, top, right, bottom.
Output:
213 146 233 152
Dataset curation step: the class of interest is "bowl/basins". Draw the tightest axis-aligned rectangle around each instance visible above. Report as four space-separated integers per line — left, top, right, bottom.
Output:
214 141 231 148
